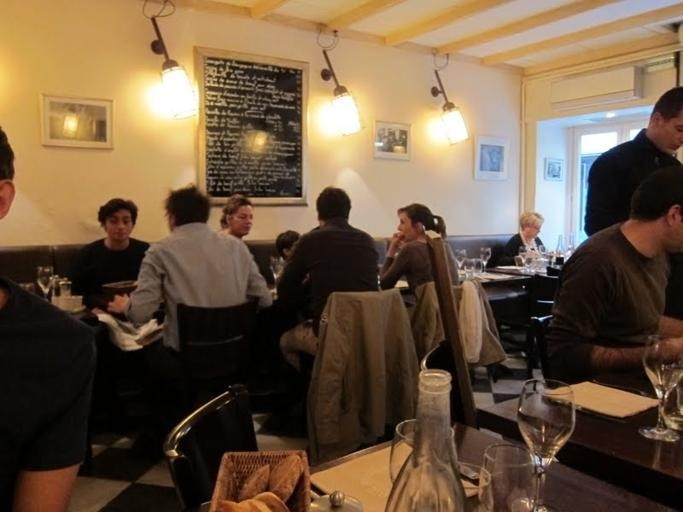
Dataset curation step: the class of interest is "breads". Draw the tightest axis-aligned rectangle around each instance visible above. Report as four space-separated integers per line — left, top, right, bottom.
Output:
215 453 305 512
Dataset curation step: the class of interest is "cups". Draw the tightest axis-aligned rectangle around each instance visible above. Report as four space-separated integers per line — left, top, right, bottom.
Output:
389 418 458 487
661 378 682 432
463 258 474 278
479 445 537 512
59 282 72 311
452 460 493 512
69 295 83 310
472 259 482 278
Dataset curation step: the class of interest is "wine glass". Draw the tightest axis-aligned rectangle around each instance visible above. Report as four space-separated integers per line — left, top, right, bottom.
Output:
518 245 548 272
480 248 491 277
453 248 466 277
637 335 682 444
270 256 283 293
37 266 53 302
515 378 575 512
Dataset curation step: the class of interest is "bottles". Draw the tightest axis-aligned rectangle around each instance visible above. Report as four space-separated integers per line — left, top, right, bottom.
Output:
384 368 466 512
565 231 575 259
555 234 565 264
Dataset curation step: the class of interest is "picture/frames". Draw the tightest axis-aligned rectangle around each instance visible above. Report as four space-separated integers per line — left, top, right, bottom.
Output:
373 119 412 161
473 134 510 180
545 158 564 181
40 94 114 150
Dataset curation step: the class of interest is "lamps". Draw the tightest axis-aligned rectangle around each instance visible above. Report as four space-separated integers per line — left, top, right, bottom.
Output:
141 0 199 120
317 23 362 133
431 49 470 146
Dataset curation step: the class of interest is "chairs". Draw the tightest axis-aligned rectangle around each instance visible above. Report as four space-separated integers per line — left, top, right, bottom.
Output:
529 262 570 394
161 384 258 512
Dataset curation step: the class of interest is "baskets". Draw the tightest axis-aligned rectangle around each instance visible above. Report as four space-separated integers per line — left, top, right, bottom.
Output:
210 450 312 512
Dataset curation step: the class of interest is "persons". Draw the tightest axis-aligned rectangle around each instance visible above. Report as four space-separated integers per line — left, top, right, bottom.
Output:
377 128 408 151
75 196 151 291
378 202 460 294
584 84 683 320
276 187 381 373
267 229 309 285
214 193 256 245
539 167 683 391
498 210 556 265
0 129 98 512
105 186 276 351
